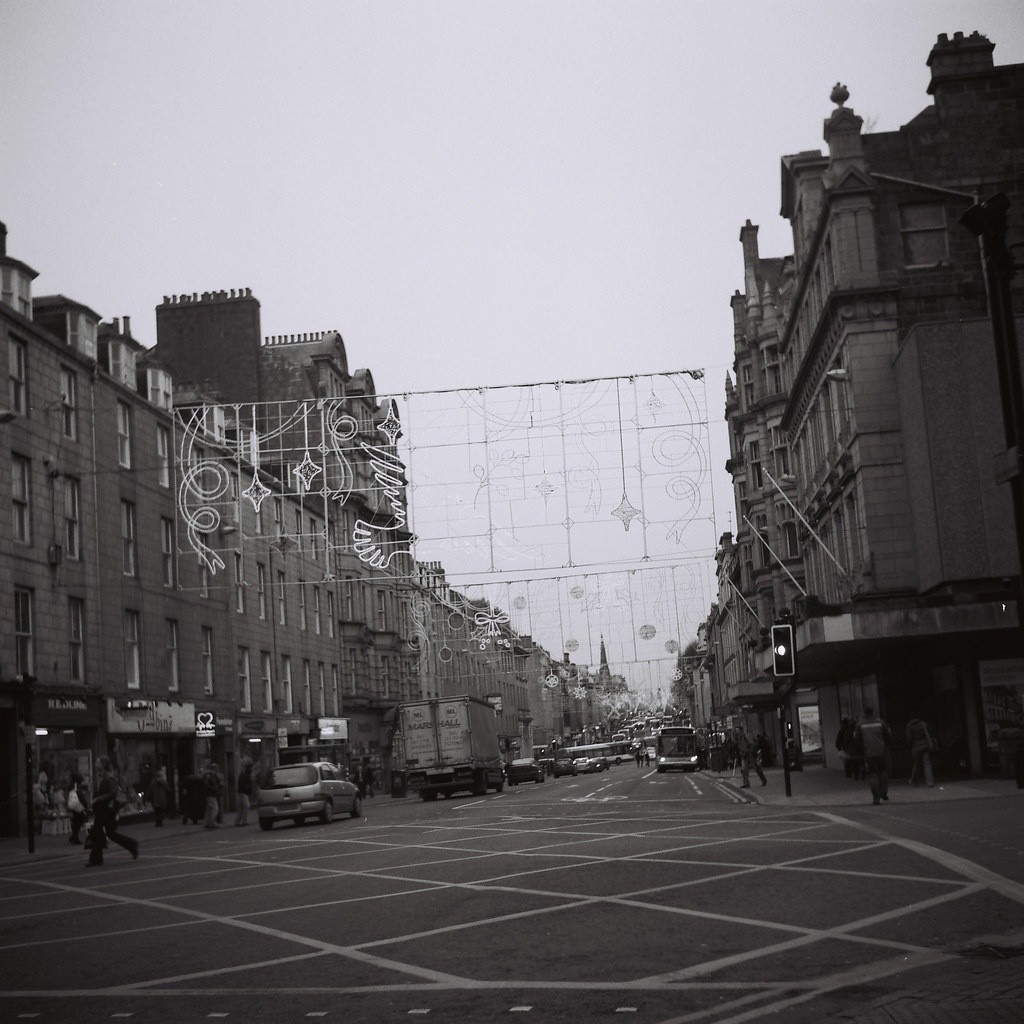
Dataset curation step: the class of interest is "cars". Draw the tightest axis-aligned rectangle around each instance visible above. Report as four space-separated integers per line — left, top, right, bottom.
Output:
505 758 545 786
612 714 674 747
538 758 606 779
257 761 364 832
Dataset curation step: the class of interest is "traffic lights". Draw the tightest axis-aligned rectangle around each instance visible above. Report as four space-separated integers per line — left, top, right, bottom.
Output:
774 624 796 678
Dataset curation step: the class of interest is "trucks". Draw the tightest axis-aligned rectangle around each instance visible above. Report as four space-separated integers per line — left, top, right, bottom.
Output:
399 696 510 802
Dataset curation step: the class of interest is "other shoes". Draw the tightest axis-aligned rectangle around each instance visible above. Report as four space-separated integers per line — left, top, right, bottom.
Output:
129 839 138 860
881 793 888 801
68 836 81 845
873 797 880 805
85 862 103 867
761 780 767 785
740 785 749 788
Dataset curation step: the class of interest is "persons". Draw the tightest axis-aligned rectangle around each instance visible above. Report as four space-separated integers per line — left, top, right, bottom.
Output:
907 710 934 788
69 775 88 845
837 716 864 781
636 751 650 768
696 726 770 788
85 755 139 867
353 763 376 799
145 763 258 830
998 720 1024 789
854 707 893 806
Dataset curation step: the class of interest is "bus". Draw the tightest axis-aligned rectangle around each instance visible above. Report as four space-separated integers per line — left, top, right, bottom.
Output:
656 726 699 772
558 740 636 768
643 736 656 762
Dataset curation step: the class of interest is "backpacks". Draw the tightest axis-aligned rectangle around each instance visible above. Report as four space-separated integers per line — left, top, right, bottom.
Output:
68 783 85 813
103 777 128 808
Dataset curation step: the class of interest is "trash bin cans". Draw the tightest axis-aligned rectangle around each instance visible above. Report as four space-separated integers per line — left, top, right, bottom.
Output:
390 769 407 799
709 745 724 772
998 728 1024 780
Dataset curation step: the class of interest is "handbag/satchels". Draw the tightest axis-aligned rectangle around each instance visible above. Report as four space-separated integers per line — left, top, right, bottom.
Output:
84 825 108 849
